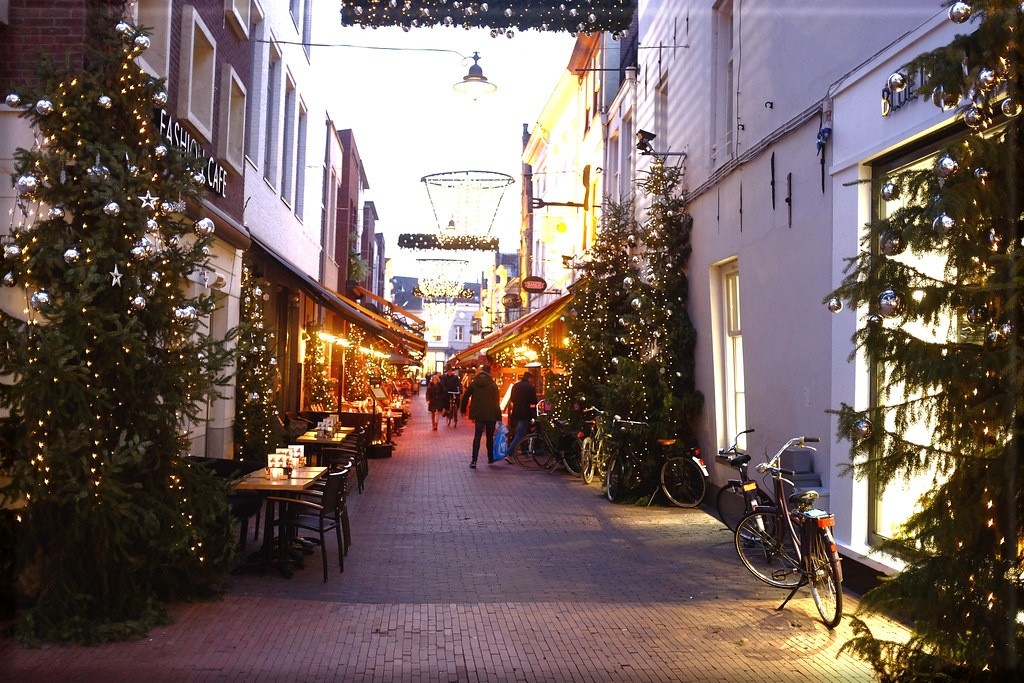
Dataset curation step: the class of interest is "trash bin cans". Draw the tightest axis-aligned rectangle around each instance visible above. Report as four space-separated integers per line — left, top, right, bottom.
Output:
285 411 309 446
772 469 829 559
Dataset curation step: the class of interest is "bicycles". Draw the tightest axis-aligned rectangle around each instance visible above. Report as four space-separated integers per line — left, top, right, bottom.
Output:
446 391 461 429
604 415 711 509
715 428 778 566
733 437 846 629
514 398 618 484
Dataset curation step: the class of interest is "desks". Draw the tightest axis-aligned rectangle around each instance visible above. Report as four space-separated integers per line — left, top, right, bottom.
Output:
382 411 403 450
225 466 329 578
296 426 355 466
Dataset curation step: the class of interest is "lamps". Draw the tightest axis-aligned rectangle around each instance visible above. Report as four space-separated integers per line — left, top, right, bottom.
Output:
415 259 469 298
248 40 498 102
420 170 516 240
421 302 457 336
637 129 687 156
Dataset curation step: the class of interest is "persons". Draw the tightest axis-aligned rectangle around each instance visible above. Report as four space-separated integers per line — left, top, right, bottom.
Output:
504 371 539 464
424 364 464 431
459 365 503 469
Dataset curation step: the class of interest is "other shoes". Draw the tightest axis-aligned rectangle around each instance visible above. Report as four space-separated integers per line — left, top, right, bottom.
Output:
523 454 534 462
504 455 515 464
488 461 495 465
470 462 476 468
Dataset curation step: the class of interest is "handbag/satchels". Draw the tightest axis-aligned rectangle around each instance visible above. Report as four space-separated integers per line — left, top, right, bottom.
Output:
493 425 508 461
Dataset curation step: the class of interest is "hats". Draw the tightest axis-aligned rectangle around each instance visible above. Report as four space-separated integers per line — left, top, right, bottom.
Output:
445 364 452 371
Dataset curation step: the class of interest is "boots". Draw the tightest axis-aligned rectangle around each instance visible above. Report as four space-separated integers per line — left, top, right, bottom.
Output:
432 423 437 430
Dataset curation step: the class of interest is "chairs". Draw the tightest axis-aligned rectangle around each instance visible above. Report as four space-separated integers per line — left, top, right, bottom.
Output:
182 455 266 550
285 410 317 438
412 383 419 396
323 420 370 494
266 461 351 583
